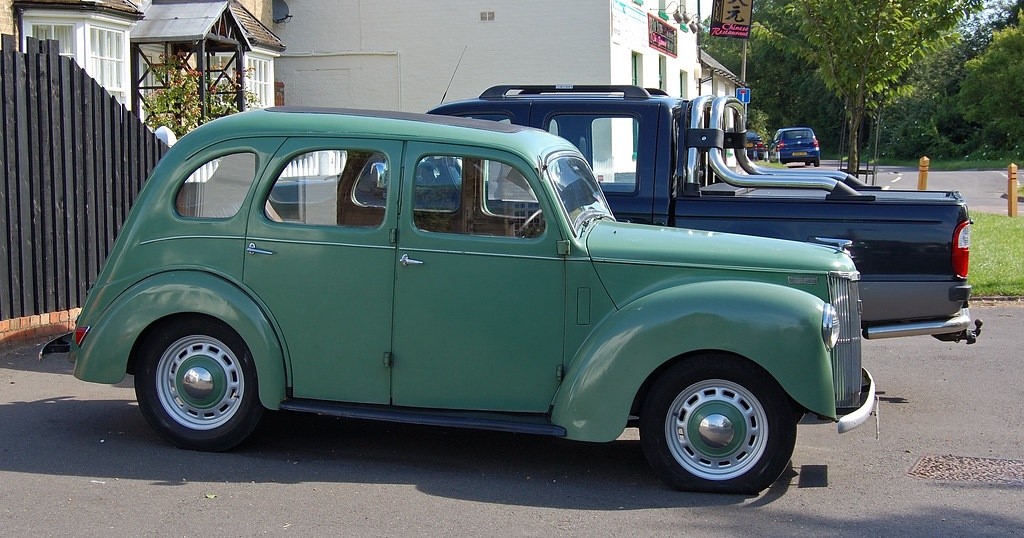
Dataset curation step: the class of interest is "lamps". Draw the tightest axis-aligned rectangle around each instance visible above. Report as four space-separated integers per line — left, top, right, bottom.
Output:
650 1 704 34
694 62 702 80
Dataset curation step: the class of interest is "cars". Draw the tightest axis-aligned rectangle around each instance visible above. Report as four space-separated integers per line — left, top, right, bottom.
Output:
744 129 765 160
771 127 820 166
37 105 876 496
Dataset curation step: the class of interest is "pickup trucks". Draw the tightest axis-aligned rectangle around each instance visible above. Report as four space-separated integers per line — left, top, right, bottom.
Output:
266 83 985 346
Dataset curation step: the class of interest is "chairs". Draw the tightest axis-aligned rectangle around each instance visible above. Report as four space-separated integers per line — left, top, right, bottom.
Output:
442 155 467 195
